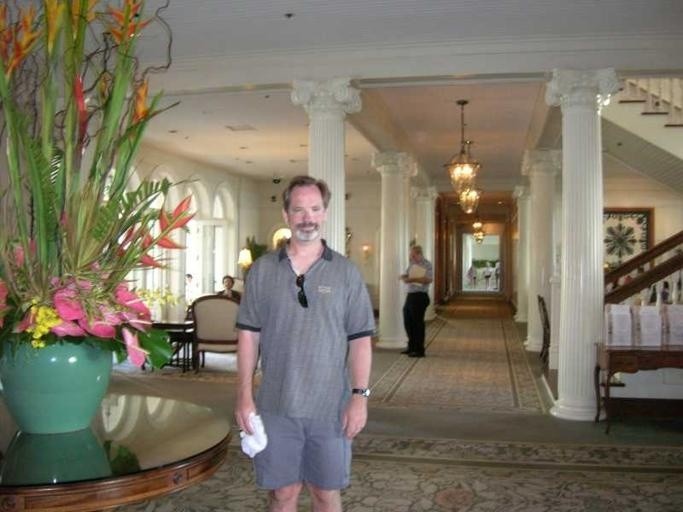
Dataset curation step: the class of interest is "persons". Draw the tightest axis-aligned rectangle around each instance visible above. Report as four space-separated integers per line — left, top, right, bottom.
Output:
216 275 240 300
233 174 378 511
468 264 476 288
493 262 500 289
482 261 492 289
396 244 433 357
186 273 200 305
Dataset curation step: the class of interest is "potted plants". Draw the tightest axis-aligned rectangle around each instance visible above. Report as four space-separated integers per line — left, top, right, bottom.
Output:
603 207 656 279
1 428 112 486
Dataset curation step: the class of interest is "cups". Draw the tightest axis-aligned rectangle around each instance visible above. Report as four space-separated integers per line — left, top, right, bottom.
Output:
297 274 308 307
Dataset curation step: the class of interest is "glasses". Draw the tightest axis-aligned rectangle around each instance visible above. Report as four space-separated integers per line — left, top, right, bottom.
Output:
445 100 485 244
239 248 253 273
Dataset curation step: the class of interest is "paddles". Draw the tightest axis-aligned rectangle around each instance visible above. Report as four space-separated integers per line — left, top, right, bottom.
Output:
109 348 263 386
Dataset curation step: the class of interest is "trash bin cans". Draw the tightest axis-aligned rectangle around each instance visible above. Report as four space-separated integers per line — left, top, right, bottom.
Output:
351 387 371 397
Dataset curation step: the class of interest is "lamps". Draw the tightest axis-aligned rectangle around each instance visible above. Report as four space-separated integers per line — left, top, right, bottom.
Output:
192 296 262 374
537 296 551 363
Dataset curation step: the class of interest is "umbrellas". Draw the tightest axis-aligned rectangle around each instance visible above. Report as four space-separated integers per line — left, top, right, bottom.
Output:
401 350 424 357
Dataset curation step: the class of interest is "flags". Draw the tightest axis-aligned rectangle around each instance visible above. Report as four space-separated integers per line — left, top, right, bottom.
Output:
1 333 114 436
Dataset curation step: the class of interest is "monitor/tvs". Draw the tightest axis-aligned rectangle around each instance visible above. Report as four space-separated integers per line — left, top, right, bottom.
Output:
408 264 427 287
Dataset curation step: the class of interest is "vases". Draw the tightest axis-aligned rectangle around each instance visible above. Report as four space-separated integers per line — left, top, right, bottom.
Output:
0 0 198 369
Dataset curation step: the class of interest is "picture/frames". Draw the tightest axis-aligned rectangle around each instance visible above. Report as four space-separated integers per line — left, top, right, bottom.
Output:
1 394 233 511
593 343 683 433
